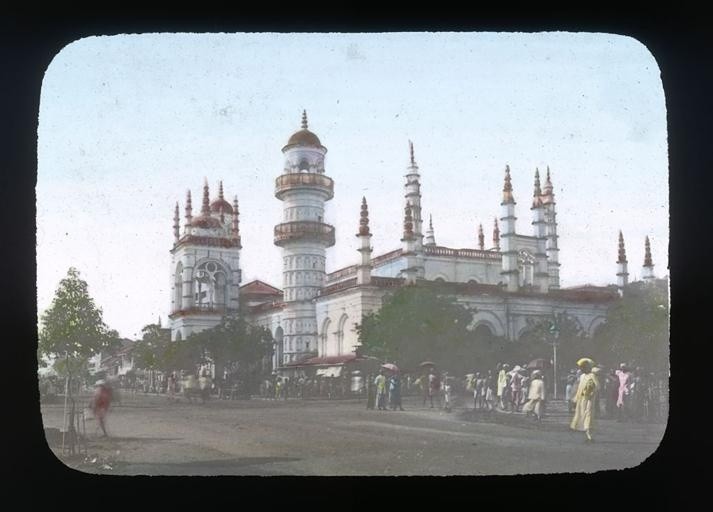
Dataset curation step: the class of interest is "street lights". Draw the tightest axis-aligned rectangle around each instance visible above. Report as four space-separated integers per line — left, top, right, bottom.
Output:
549 321 560 400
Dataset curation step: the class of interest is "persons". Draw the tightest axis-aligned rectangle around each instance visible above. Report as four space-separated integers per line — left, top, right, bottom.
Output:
367 358 556 425
91 379 113 436
566 357 656 444
222 370 373 403
115 364 213 407
